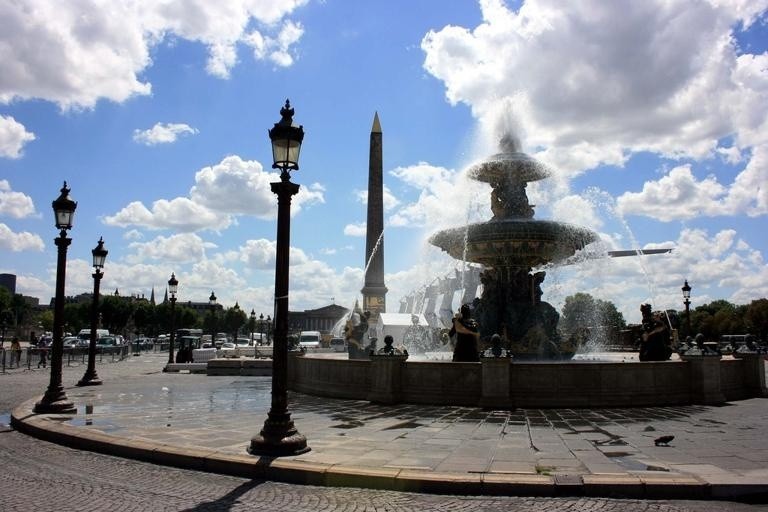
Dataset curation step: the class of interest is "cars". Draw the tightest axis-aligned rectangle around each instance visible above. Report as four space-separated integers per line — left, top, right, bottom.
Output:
202 332 269 347
131 336 154 352
155 333 179 349
219 343 241 358
39 329 128 356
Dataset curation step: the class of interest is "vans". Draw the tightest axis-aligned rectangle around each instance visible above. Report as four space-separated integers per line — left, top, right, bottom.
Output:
297 330 321 348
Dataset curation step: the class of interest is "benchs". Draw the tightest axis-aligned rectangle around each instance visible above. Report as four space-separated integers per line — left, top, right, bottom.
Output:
167 363 208 373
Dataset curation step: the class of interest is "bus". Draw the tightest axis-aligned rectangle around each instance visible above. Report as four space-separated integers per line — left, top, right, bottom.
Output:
177 328 203 347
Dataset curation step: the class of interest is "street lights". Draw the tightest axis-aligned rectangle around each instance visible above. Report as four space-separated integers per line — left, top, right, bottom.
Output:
233 300 240 344
267 314 271 346
259 313 264 346
682 279 692 328
162 271 181 373
78 235 108 386
245 99 311 456
209 291 218 347
250 309 255 346
31 179 79 414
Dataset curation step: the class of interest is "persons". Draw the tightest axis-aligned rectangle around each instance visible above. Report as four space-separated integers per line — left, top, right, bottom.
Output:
446 304 481 362
37 341 47 368
9 338 20 369
639 304 668 361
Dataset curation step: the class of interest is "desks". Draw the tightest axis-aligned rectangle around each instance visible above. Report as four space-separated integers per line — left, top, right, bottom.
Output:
192 347 218 363
